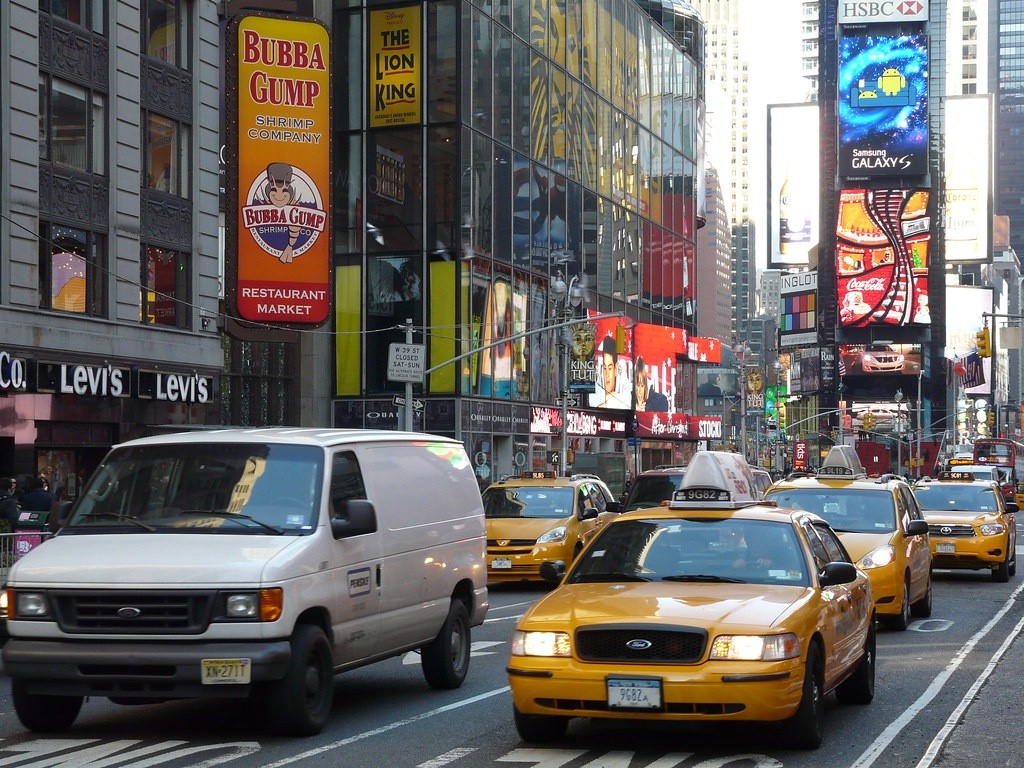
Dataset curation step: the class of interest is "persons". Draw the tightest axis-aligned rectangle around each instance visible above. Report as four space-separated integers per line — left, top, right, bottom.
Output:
774 465 790 482
792 465 817 473
0 464 67 531
589 336 631 409
697 373 721 395
631 356 668 412
858 428 872 441
736 525 786 571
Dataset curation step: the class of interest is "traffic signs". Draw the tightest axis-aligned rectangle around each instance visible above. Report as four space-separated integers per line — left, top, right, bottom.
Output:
392 395 426 414
554 396 579 408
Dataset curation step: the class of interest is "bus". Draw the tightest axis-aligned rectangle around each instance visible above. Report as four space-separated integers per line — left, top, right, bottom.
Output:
973 438 1024 509
944 453 974 471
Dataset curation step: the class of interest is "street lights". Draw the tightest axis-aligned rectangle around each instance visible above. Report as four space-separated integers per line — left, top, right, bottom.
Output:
905 400 912 479
735 341 753 459
773 361 784 475
1005 424 1008 438
549 270 582 476
895 389 904 477
913 365 926 481
756 421 764 462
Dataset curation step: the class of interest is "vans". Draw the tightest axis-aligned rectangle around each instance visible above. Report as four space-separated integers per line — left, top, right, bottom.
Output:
620 464 774 539
952 466 999 484
2 426 491 736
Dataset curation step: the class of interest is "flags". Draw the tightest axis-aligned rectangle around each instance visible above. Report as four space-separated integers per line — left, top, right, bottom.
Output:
961 352 985 388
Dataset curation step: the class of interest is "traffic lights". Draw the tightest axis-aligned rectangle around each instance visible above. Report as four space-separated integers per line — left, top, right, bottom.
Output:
547 449 560 465
986 429 991 438
831 432 836 439
800 432 804 439
989 412 994 425
977 327 991 357
869 413 876 430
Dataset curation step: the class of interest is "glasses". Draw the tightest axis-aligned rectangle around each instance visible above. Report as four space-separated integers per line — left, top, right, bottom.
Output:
12 482 16 484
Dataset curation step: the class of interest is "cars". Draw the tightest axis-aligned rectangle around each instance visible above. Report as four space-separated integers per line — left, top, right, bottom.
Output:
508 449 878 753
902 471 1017 579
477 475 617 585
760 443 934 630
